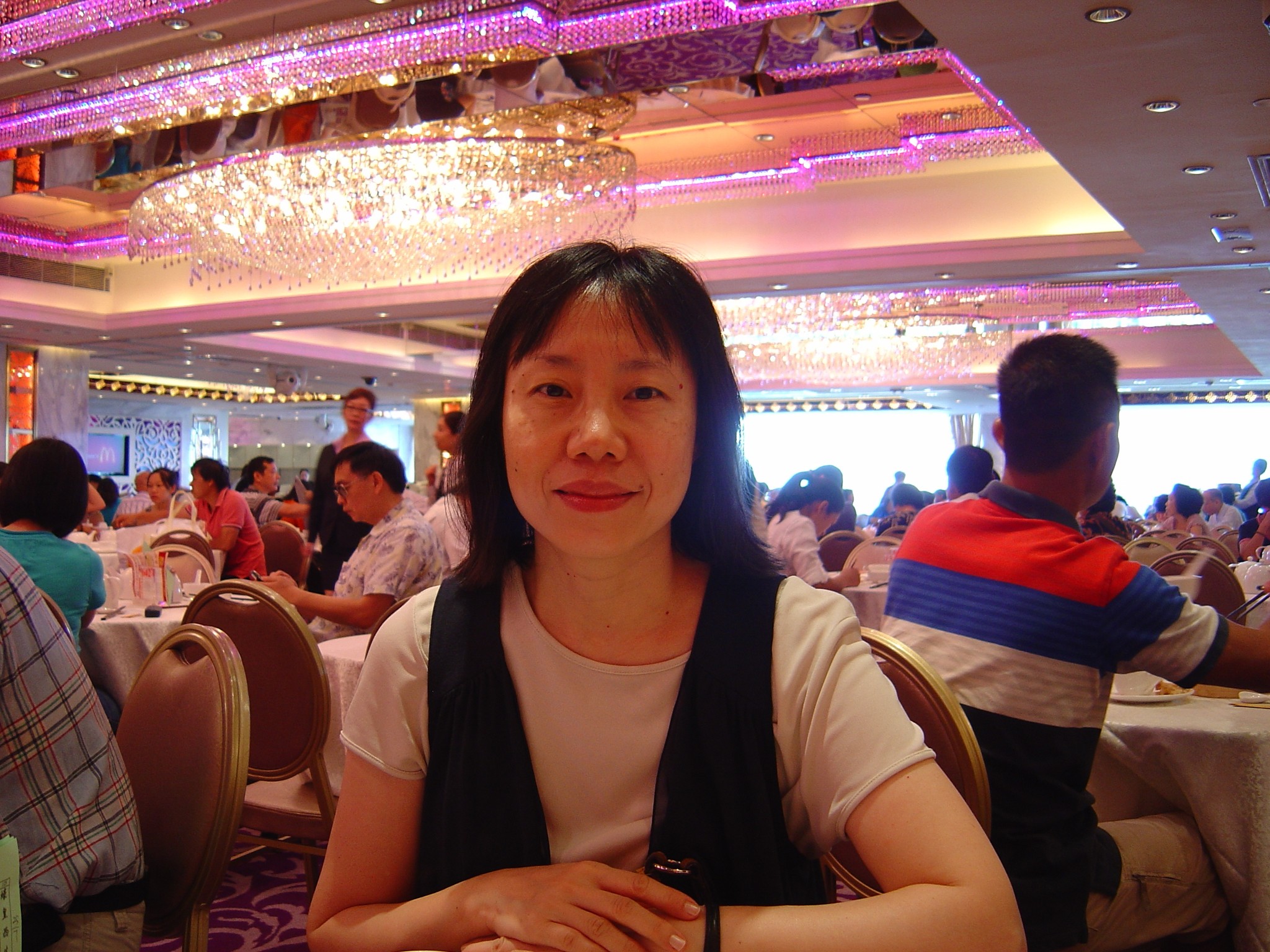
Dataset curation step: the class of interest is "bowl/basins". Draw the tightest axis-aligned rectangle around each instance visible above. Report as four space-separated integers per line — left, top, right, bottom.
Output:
867 563 890 582
183 582 211 598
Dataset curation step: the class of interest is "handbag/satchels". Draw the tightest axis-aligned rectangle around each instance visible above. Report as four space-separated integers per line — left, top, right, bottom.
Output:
144 490 208 550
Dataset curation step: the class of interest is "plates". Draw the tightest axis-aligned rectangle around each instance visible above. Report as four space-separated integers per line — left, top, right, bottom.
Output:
1110 674 1194 700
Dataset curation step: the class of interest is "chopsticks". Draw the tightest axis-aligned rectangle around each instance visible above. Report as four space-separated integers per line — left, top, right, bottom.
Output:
1225 590 1270 622
101 606 125 620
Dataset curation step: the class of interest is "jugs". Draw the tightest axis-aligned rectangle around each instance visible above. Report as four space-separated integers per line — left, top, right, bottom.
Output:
1243 559 1270 594
66 529 97 546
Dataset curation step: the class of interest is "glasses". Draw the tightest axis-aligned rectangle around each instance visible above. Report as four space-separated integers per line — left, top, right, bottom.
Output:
344 404 372 415
334 472 369 497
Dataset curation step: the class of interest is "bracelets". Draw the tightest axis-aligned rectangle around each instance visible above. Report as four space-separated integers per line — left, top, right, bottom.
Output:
704 901 721 952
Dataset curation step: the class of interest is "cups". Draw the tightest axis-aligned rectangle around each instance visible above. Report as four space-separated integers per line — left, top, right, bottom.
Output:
1227 556 1257 588
96 577 120 614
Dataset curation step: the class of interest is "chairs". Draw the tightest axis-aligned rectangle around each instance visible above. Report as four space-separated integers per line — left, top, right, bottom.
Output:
815 525 1246 899
258 520 307 587
183 575 338 903
150 543 218 588
150 529 215 570
114 622 250 952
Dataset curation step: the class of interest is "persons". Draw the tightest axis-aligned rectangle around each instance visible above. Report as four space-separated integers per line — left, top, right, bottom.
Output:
764 471 860 592
884 333 1270 952
307 243 1034 952
0 386 1270 952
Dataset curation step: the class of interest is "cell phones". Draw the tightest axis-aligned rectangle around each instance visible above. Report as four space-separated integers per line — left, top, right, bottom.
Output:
250 570 264 581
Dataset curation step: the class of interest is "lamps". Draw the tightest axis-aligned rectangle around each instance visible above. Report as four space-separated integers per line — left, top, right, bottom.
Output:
0 1 1214 388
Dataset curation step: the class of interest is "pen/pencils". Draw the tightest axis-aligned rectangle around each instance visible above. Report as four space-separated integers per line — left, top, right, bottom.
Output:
849 558 858 568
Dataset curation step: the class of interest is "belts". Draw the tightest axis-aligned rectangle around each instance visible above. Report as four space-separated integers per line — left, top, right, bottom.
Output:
62 879 146 914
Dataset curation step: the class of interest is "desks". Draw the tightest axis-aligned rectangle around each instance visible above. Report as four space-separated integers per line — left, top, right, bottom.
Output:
87 603 186 711
842 573 1270 952
313 633 371 797
